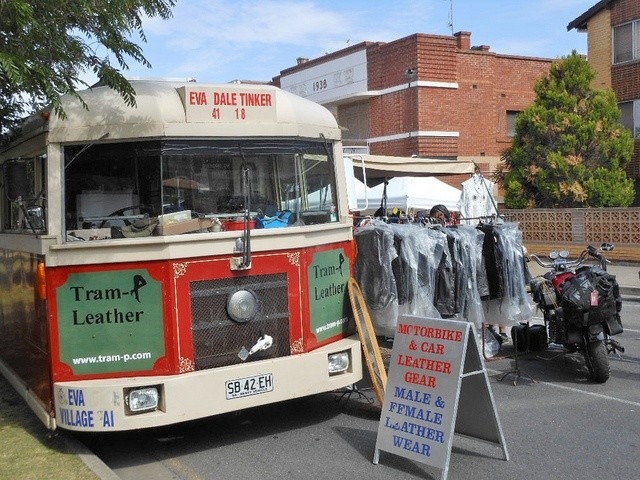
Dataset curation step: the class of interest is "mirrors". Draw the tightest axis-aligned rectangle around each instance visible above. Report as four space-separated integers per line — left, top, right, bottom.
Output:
347 277 387 404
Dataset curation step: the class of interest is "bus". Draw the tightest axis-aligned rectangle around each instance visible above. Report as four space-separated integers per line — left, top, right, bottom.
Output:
0 75 364 441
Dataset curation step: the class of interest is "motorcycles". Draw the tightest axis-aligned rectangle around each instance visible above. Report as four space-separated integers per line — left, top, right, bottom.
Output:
526 242 625 383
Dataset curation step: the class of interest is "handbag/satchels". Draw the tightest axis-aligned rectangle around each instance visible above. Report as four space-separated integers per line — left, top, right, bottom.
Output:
511 321 549 350
559 265 615 312
529 276 559 313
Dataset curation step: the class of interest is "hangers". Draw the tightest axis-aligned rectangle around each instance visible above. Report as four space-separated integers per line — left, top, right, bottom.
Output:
365 215 497 228
461 167 496 187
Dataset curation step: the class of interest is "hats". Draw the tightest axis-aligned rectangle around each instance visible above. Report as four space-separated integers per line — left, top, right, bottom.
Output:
436 204 451 219
